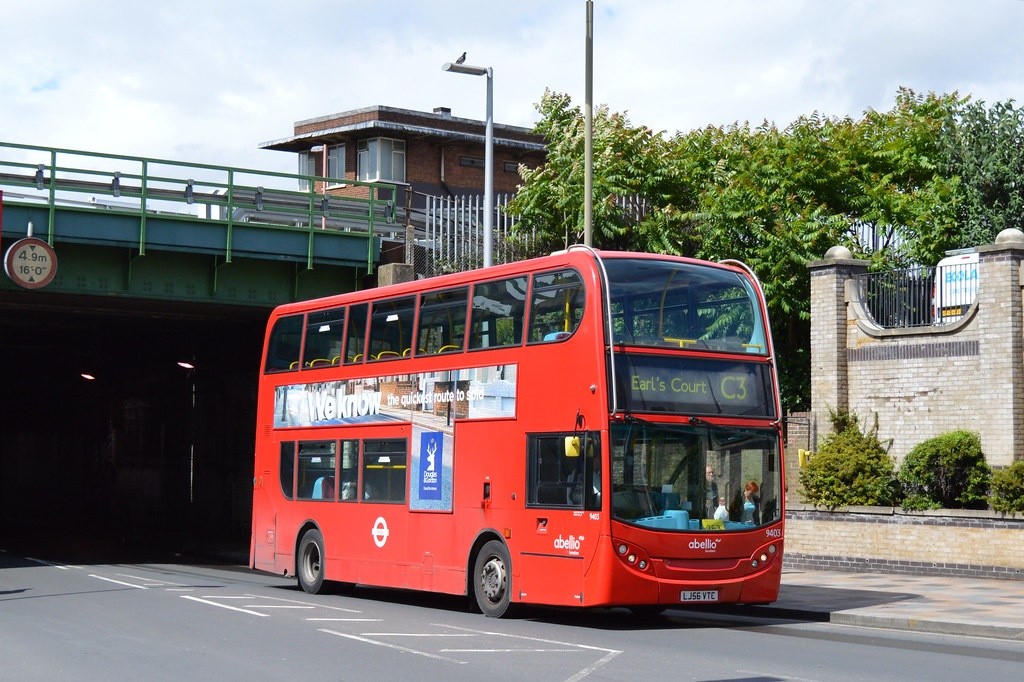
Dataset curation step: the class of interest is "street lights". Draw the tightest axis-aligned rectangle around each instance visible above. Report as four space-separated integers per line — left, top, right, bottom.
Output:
444 64 494 267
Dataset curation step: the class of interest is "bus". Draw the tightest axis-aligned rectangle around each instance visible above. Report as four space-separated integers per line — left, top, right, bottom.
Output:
248 243 816 619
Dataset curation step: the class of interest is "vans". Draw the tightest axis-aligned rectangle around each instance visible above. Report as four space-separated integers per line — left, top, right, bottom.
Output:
930 248 979 326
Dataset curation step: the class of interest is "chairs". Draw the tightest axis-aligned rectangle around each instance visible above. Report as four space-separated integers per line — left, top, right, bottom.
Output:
289 331 603 508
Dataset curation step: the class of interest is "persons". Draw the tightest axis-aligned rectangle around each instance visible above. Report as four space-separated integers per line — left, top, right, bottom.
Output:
94 462 121 529
704 466 720 519
729 481 760 525
